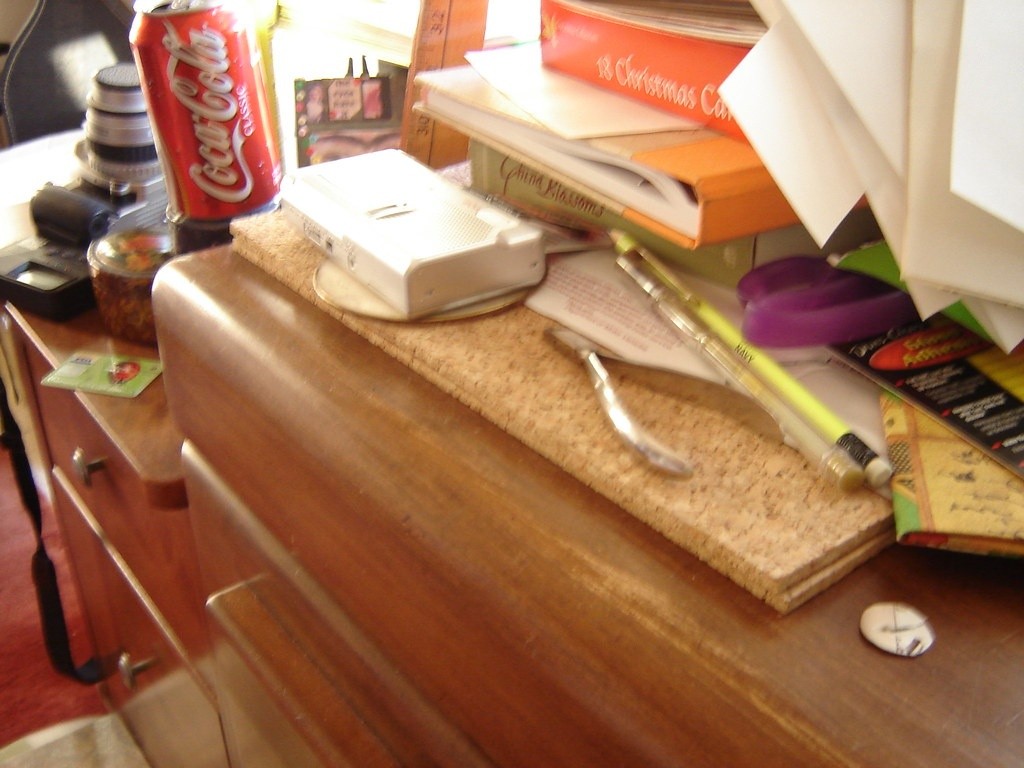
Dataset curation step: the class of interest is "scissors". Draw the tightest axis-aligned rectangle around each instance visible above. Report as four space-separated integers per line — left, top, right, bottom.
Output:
734 245 920 349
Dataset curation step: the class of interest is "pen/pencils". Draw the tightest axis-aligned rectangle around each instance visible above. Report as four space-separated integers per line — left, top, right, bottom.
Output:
608 227 895 489
611 252 866 497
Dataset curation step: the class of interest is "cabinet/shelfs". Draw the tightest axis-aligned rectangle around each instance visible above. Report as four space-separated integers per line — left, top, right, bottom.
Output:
0 244 1022 768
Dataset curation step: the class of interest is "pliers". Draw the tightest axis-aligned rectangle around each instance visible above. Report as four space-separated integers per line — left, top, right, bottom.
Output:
542 329 785 482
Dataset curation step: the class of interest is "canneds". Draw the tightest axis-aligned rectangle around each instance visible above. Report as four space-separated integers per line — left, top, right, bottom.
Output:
127 0 284 223
